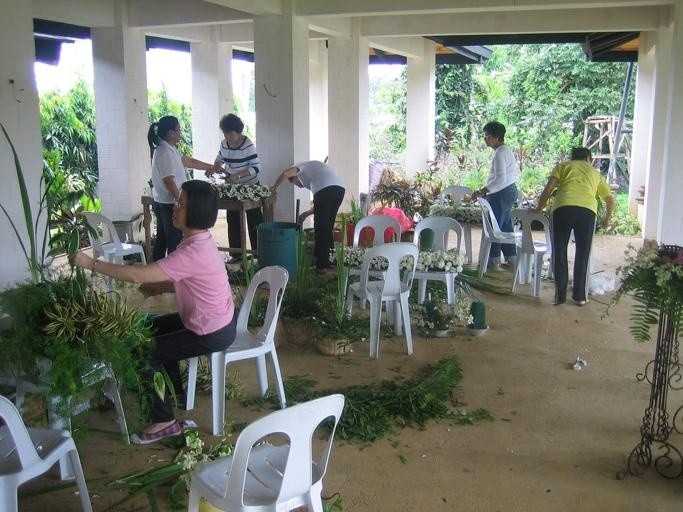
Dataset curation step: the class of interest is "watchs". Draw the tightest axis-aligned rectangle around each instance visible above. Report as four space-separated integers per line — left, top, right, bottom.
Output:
92 260 101 272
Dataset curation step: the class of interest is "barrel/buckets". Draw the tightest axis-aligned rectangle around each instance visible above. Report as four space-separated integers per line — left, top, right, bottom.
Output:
257 222 300 289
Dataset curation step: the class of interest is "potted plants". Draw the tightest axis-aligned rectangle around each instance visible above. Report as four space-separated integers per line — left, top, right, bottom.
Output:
0 120 178 464
222 212 394 357
334 190 367 250
468 301 490 338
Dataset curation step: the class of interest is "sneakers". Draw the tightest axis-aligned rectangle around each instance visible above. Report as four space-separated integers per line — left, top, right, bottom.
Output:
225 256 238 263
130 420 181 445
318 267 326 274
169 392 186 407
576 299 590 305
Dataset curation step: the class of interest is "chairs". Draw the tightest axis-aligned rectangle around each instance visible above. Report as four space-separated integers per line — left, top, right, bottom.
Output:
334 213 463 359
440 185 549 298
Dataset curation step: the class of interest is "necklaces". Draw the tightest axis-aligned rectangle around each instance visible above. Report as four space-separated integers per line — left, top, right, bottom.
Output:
228 136 240 167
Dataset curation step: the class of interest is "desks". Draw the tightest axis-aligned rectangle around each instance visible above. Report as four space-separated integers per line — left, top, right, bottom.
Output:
140 196 273 287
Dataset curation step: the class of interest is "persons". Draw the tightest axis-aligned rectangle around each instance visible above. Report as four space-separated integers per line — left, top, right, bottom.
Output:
471 120 519 271
146 115 228 263
525 146 616 306
67 179 238 446
268 160 345 275
204 114 264 264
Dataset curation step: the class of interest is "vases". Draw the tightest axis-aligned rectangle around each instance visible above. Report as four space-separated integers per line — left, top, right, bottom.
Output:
616 244 682 481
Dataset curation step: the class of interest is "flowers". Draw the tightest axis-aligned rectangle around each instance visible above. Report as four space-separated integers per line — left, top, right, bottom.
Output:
146 177 272 201
328 246 473 340
429 204 547 231
598 238 683 345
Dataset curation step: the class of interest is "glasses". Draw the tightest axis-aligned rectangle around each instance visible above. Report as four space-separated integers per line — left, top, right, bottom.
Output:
172 198 184 208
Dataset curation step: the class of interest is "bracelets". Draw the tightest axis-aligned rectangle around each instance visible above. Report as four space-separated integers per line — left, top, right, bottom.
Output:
159 283 163 296
236 171 241 181
478 188 489 196
